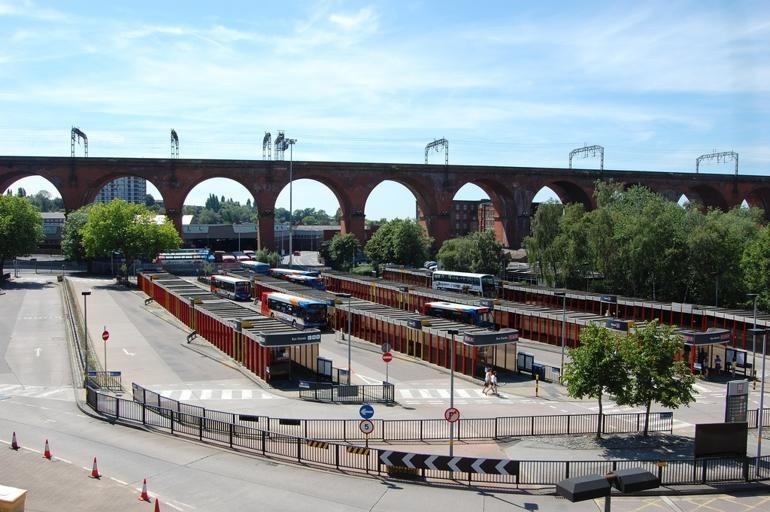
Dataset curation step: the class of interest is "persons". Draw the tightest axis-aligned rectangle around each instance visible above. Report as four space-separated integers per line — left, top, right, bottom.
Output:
714 354 723 375
482 367 495 393
731 357 737 376
699 347 705 375
699 351 709 376
485 370 498 395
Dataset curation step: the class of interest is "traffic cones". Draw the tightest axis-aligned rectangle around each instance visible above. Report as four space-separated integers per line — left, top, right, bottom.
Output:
88 456 102 478
137 478 160 512
42 438 53 458
10 432 18 450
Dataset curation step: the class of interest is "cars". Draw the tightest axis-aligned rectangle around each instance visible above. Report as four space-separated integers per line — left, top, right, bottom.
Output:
424 261 438 271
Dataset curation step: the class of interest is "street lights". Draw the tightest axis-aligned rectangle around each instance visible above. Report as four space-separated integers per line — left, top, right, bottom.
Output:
748 327 769 478
556 467 660 512
286 137 298 268
334 292 352 385
82 291 92 387
745 293 760 358
553 292 566 385
447 330 459 457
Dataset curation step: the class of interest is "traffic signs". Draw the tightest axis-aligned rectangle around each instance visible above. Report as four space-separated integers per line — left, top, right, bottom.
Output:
378 449 519 476
360 420 374 433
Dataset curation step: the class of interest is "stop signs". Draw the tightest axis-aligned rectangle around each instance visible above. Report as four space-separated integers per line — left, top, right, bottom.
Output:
383 353 392 363
101 331 109 340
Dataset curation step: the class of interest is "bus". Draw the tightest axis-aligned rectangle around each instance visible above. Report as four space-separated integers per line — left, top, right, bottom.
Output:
423 301 496 330
210 250 327 332
432 271 495 298
